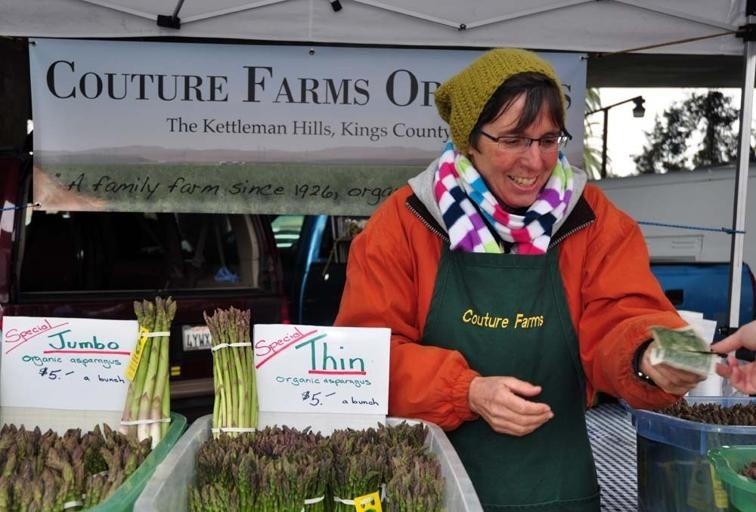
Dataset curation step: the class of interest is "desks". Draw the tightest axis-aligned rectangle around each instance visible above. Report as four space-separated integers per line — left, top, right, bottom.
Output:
584 96 646 179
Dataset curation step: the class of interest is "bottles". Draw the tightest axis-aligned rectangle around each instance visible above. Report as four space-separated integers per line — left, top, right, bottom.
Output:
434 48 567 154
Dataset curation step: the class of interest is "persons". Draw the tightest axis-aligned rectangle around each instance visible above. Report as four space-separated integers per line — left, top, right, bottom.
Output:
333 43 710 511
710 319 756 398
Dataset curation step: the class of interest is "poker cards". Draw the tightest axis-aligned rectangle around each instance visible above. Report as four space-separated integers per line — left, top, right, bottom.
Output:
654 398 756 479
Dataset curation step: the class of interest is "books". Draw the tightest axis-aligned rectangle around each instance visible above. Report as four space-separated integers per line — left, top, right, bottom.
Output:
618 394 756 512
2 408 187 511
706 444 756 511
131 413 482 512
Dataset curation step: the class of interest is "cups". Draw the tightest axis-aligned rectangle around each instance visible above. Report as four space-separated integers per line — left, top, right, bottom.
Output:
478 126 573 157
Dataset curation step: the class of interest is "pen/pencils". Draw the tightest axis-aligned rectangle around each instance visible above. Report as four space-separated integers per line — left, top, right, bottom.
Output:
281 215 370 326
7 212 291 412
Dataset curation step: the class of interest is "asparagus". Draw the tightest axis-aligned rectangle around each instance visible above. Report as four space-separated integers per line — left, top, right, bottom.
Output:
119 296 175 450
187 420 445 510
203 305 258 437
0 422 153 512
654 398 756 479
